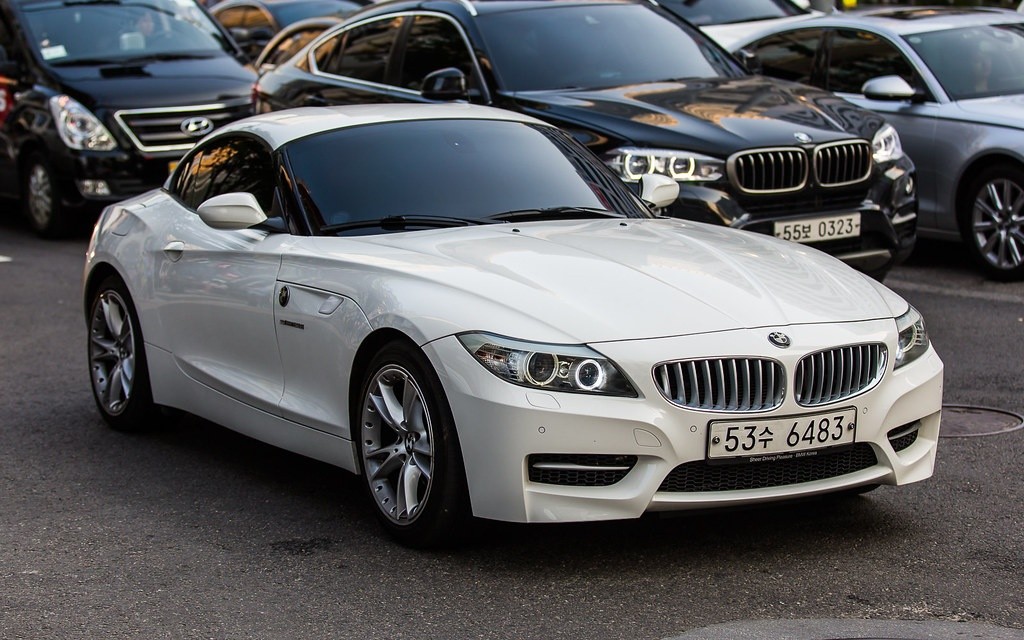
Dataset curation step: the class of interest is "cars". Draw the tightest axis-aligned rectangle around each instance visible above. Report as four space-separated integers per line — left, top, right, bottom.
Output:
723 6 1023 283
656 1 824 52
194 1 365 77
83 103 943 551
250 0 918 284
0 1 261 236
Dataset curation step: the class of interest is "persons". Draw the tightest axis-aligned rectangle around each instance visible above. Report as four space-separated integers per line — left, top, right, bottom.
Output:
113 6 155 51
